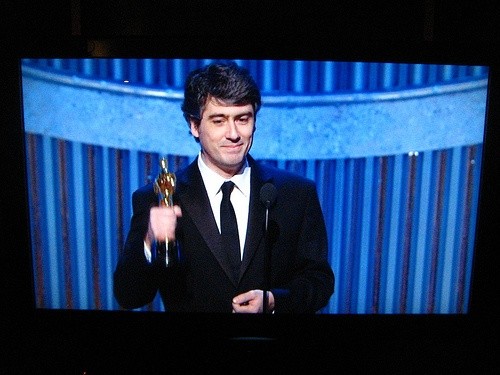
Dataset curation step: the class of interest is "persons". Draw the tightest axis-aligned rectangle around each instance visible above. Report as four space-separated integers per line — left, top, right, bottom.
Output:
113 62 335 314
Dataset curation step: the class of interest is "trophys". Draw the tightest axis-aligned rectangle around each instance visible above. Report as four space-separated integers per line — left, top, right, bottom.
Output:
154 157 176 241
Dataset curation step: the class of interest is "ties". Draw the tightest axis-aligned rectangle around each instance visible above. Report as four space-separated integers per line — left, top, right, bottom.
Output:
220 181 241 267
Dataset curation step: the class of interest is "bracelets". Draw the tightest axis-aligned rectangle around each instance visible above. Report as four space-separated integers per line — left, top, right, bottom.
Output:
263 289 268 314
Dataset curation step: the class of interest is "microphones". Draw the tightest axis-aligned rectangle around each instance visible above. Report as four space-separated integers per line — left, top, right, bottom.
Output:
260 183 278 313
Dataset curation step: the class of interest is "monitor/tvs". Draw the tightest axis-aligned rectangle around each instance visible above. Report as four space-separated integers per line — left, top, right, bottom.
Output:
7 35 499 336
